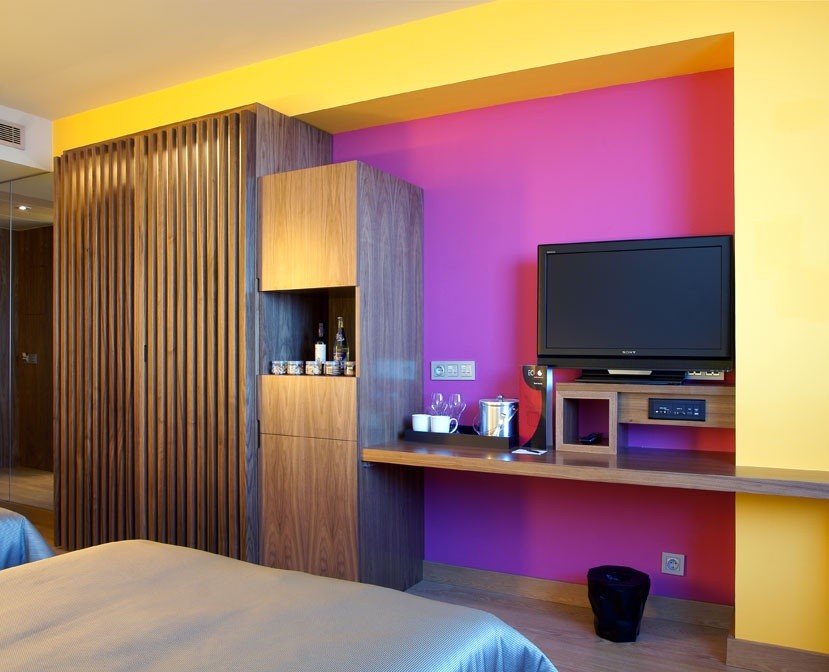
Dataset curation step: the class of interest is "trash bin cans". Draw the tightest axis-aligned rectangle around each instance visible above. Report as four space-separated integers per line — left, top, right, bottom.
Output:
586 564 651 642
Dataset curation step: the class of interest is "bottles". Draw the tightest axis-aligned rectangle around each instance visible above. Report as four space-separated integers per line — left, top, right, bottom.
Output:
332 316 349 375
314 323 328 371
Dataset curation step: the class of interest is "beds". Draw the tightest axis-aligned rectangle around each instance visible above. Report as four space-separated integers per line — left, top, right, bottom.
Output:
0 537 559 672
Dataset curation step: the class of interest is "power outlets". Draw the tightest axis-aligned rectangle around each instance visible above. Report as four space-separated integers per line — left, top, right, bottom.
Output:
430 360 475 380
662 552 684 576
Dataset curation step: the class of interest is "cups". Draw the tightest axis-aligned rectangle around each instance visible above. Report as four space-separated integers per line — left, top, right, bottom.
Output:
305 361 322 375
271 360 287 375
322 360 341 376
286 361 304 375
430 416 458 434
411 413 432 432
343 361 356 376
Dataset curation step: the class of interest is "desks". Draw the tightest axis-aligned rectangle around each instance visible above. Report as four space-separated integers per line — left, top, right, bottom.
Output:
362 439 829 501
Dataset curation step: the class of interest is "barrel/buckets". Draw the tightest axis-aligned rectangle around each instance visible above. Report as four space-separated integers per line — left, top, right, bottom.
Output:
472 395 519 438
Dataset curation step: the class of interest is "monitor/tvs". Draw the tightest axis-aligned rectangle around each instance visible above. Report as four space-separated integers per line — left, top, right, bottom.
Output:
537 235 735 385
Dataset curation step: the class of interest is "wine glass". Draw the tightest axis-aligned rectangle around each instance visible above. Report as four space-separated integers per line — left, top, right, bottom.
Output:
448 393 466 434
429 392 449 417
